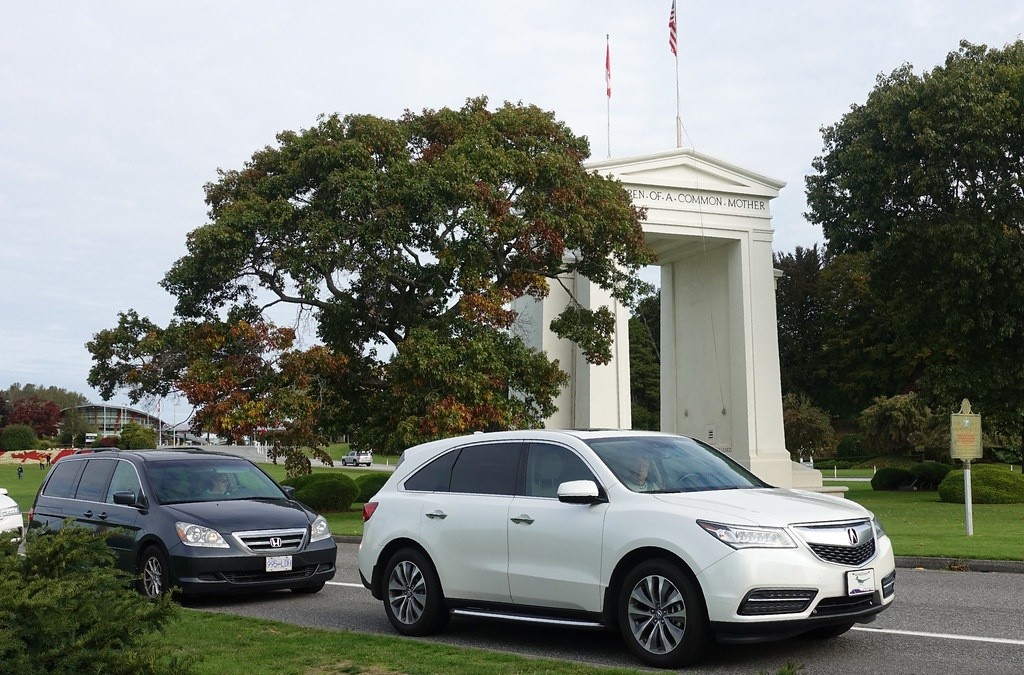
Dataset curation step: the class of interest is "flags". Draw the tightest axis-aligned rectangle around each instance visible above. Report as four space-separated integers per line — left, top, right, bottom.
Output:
669 0 677 58
606 43 611 98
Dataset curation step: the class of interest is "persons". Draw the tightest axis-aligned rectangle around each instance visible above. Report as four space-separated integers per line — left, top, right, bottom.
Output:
46 453 52 467
202 472 231 495
38 454 46 470
17 465 23 480
622 457 660 492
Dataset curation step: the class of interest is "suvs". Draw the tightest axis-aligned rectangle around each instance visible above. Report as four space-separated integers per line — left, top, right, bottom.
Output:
341 450 374 467
355 426 899 668
24 445 337 604
